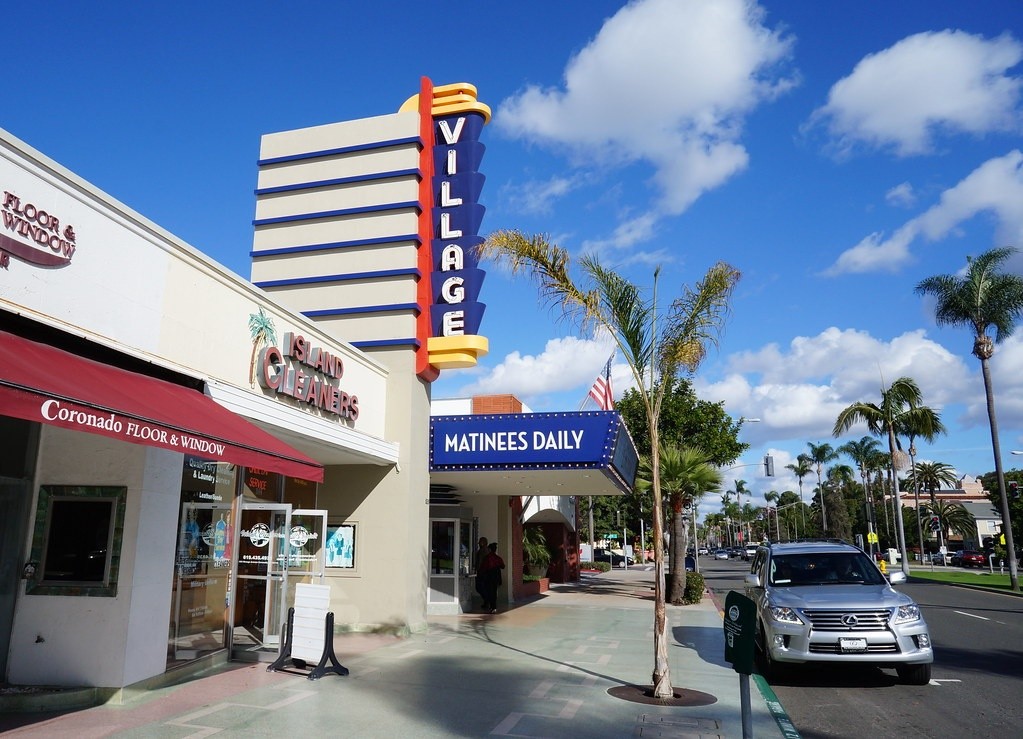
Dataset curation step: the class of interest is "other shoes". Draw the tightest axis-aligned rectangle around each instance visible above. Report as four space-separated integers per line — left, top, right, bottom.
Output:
489 608 496 613
480 604 489 611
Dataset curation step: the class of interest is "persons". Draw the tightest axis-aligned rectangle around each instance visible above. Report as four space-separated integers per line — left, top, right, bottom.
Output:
473 536 505 613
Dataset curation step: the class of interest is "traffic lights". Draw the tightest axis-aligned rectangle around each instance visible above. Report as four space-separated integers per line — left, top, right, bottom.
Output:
1008 481 1019 499
930 515 940 532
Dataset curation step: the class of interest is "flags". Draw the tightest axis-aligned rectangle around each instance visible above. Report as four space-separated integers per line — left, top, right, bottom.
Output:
588 358 613 411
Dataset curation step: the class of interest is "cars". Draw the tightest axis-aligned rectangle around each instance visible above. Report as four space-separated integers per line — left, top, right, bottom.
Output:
931 550 986 568
697 544 762 561
744 540 934 686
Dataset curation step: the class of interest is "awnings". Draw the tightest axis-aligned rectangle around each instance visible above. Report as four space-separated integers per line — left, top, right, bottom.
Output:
0 331 323 483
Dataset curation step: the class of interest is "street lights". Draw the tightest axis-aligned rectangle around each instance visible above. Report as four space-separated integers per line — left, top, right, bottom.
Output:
692 418 761 572
744 501 807 545
